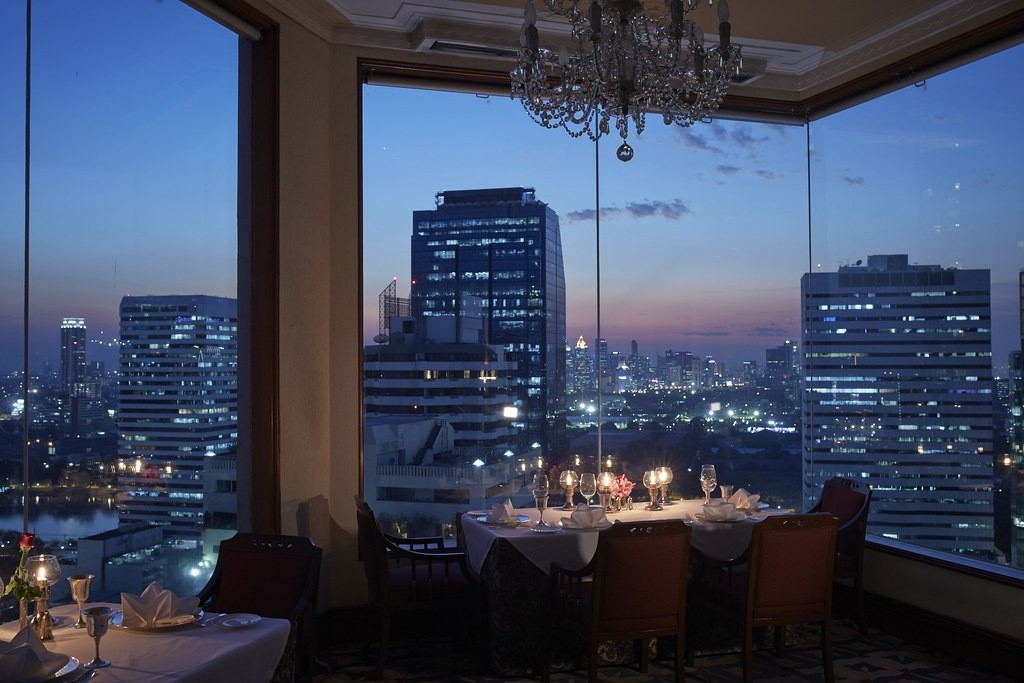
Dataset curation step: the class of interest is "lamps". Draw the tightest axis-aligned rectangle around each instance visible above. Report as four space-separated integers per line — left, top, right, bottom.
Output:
509 1 743 161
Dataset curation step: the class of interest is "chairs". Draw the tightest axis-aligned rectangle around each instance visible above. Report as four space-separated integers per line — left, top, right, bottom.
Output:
190 531 324 683
355 496 479 679
702 513 840 682
747 476 874 647
543 517 693 682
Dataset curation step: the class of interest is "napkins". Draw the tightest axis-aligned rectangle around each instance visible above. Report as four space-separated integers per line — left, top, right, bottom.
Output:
486 496 523 529
1 624 72 682
118 579 201 630
561 507 609 528
698 498 738 520
727 487 767 514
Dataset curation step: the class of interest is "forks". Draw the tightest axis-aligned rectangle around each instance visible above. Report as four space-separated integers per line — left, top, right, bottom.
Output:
685 513 698 523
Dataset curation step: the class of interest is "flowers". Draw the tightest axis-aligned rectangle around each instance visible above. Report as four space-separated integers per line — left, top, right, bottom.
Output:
3 532 42 604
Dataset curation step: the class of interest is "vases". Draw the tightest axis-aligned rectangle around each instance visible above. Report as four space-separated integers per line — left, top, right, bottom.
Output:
17 595 30 630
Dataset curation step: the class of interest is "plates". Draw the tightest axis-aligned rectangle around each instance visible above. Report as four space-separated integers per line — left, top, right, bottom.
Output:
211 614 261 628
467 509 494 515
559 521 613 530
54 656 79 677
530 527 561 532
755 503 769 511
761 508 795 516
694 513 747 521
477 515 531 522
109 609 204 628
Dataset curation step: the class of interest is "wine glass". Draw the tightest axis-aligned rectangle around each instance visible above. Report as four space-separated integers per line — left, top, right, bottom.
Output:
67 573 96 628
533 474 549 522
701 465 717 506
82 607 111 668
534 497 551 527
719 485 735 502
580 473 597 506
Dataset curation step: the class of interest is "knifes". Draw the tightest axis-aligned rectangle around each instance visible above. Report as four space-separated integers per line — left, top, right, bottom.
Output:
69 669 93 683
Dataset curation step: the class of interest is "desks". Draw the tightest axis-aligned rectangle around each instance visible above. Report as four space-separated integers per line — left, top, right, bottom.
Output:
455 496 798 682
0 586 292 683
